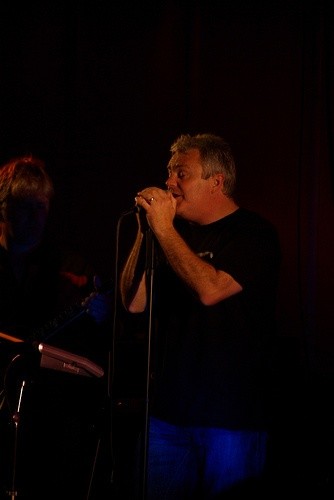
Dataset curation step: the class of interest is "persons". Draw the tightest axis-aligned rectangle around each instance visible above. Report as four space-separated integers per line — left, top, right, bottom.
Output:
119 132 265 489
0 152 113 500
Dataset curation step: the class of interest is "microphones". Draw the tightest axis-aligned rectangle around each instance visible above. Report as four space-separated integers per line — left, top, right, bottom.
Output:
123 205 144 217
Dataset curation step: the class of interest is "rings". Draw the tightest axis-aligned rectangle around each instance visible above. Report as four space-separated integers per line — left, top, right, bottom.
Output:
150 197 154 201
